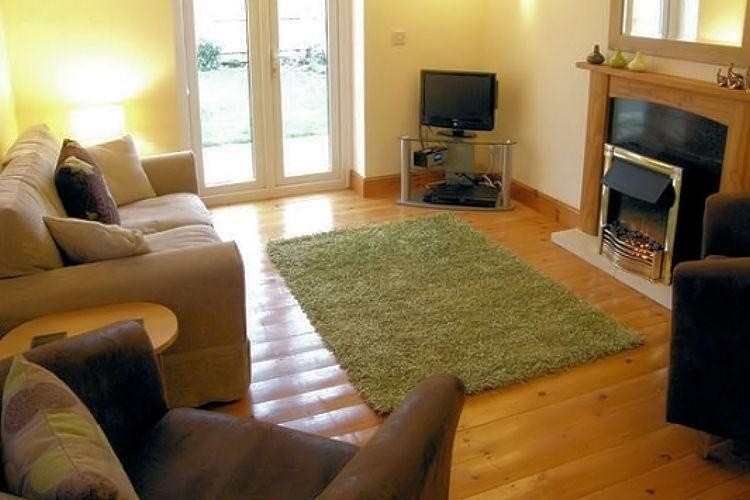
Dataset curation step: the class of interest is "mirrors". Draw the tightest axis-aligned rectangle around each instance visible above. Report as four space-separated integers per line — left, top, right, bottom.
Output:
605 0 750 70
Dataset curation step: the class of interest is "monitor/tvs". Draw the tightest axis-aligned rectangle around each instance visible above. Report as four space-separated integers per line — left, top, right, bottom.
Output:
418 68 496 138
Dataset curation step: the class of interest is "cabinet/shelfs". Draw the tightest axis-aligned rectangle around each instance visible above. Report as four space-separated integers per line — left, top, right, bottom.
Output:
397 130 518 213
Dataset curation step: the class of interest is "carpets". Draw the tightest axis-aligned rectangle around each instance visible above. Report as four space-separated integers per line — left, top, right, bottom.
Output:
267 213 642 418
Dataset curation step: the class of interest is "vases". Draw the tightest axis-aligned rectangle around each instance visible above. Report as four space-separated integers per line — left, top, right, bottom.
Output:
609 47 626 69
587 44 605 65
626 49 647 70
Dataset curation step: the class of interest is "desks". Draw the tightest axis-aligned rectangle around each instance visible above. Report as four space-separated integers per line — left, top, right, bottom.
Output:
1 301 179 405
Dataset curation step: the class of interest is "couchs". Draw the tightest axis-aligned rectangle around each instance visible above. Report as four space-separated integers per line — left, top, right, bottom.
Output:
2 118 248 409
0 318 466 500
668 190 750 464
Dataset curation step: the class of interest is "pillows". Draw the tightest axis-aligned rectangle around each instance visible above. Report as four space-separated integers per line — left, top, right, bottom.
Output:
60 137 91 161
85 135 154 206
1 350 143 500
55 154 122 224
43 215 150 262
